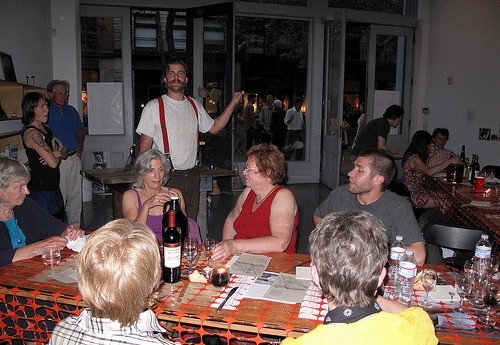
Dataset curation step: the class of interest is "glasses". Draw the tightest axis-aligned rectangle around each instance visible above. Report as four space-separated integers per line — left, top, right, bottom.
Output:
244 166 260 172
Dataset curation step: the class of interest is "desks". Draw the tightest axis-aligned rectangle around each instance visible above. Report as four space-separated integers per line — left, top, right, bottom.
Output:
429 175 500 255
80 163 236 243
0 243 500 345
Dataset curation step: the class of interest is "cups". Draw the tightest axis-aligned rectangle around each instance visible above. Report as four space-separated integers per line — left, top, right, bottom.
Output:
475 176 484 190
211 267 230 286
41 245 61 266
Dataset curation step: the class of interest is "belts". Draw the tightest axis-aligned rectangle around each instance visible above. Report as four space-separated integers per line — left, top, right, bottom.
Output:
68 151 77 156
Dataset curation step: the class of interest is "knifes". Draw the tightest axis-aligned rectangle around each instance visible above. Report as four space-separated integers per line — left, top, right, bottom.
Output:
216 287 239 311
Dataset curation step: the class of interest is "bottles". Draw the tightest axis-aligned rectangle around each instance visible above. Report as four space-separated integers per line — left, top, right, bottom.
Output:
162 196 189 253
387 235 406 279
399 251 417 301
163 210 181 284
460 144 480 183
475 235 491 259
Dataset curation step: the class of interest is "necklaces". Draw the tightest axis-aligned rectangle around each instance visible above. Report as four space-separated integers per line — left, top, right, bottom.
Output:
255 183 278 205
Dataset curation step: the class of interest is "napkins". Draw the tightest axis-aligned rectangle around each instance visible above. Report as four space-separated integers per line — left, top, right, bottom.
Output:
296 267 313 281
64 235 86 252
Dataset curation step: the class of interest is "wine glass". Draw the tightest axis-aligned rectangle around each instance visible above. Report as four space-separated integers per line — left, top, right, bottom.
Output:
483 170 495 186
184 236 197 268
208 156 216 171
182 240 199 274
202 238 215 271
387 252 500 325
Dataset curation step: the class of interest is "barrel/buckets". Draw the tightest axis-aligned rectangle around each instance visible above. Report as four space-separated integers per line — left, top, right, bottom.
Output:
446 163 464 183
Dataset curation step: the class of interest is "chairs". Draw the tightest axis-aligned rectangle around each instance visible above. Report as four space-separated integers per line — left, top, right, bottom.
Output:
421 223 488 268
482 165 500 179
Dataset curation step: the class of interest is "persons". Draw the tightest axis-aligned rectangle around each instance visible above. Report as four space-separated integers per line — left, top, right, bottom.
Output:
0 155 84 268
279 210 438 345
401 130 464 214
211 143 299 262
352 106 404 161
121 148 187 245
135 59 245 222
45 79 86 232
240 92 364 158
427 128 458 178
312 149 428 265
48 218 182 345
19 92 70 222
